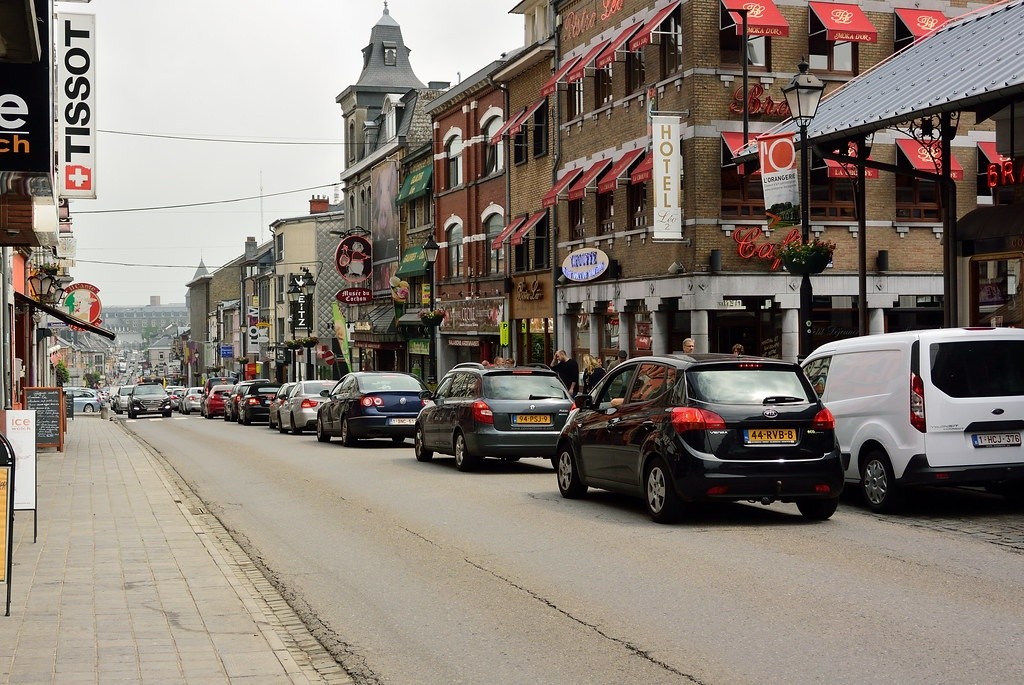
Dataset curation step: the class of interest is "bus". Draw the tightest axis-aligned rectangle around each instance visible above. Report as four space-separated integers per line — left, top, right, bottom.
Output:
137 375 165 390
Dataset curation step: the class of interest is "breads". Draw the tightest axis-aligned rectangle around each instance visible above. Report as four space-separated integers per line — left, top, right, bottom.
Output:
389 276 410 289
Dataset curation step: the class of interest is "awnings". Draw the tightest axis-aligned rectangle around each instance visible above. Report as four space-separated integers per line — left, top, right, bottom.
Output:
14 291 115 340
395 246 429 278
721 131 763 175
808 0 878 43
542 149 653 208
895 138 963 180
894 7 946 48
823 141 880 178
492 99 546 144
492 210 547 249
977 142 1011 177
719 0 789 37
395 163 433 206
540 1 682 97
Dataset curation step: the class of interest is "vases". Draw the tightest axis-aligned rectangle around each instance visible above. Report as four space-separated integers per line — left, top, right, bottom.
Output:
195 375 199 377
240 360 248 364
213 369 219 372
783 255 828 275
303 342 315 347
289 344 299 349
421 316 444 326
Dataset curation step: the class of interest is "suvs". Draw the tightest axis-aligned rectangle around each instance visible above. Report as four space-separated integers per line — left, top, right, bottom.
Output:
554 352 844 524
413 363 577 472
63 387 102 413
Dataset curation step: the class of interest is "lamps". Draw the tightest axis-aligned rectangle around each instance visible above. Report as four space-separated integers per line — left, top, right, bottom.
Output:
875 249 889 272
557 275 568 283
15 265 64 310
709 249 721 272
503 277 512 293
668 263 684 274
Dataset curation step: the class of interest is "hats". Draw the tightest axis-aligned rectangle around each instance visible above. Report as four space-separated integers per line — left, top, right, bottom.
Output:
617 350 627 358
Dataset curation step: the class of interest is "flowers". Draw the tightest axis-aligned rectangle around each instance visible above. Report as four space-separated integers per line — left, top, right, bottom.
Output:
210 365 221 369
301 336 319 345
194 372 201 375
238 356 249 361
417 308 447 319
775 237 838 265
284 339 302 345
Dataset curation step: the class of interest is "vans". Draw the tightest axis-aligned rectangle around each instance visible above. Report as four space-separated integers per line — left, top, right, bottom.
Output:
198 377 237 416
800 326 1024 513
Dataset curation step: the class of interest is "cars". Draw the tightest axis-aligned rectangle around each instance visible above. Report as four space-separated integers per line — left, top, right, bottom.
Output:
165 385 203 415
315 370 432 446
103 385 172 418
269 380 339 434
203 383 280 425
118 349 138 385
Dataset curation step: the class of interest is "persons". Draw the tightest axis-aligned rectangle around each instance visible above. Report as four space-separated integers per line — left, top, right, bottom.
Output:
550 350 579 399
582 354 607 394
482 356 515 367
732 344 744 355
683 338 694 353
606 350 627 373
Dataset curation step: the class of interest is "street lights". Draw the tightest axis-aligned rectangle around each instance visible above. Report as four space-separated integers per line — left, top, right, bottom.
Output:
780 55 827 362
240 321 247 380
212 337 218 376
422 234 440 379
288 284 300 383
301 274 316 380
194 349 199 387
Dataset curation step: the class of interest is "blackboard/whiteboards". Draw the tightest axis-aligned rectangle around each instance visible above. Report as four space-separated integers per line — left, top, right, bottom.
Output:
23 386 63 447
0 408 38 617
65 394 74 417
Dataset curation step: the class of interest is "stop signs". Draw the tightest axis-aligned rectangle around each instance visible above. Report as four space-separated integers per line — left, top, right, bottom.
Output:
324 351 334 365
